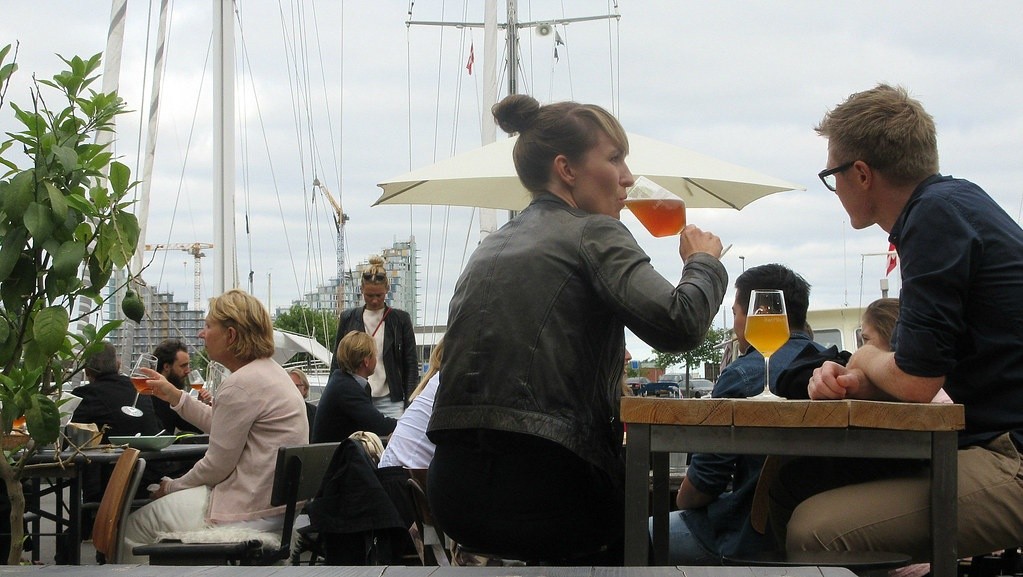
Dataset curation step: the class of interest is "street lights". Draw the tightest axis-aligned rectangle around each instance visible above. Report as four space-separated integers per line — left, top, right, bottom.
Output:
739 255 745 272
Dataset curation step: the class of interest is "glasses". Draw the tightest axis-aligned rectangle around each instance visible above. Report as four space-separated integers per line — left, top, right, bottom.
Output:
362 273 387 287
818 158 871 191
295 384 306 388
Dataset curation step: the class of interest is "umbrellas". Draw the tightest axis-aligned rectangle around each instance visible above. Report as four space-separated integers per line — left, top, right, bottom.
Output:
374 133 796 215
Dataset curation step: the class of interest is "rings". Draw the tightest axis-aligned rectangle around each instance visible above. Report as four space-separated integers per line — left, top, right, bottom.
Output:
151 389 154 395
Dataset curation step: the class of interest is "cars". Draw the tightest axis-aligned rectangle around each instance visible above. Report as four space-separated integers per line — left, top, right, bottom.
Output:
668 379 715 398
624 377 651 393
647 374 693 395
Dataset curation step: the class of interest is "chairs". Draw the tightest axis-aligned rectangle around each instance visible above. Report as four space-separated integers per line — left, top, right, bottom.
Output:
132 431 458 568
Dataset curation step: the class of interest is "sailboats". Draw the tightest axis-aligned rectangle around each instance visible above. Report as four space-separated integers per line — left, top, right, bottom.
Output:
73 0 620 369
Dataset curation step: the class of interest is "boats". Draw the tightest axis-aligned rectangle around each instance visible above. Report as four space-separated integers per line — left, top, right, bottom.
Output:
721 253 899 354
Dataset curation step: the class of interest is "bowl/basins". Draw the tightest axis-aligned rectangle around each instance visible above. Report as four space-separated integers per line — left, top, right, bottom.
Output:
108 436 180 451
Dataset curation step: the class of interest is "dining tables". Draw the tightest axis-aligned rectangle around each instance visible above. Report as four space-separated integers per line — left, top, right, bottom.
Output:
619 393 967 577
7 442 210 566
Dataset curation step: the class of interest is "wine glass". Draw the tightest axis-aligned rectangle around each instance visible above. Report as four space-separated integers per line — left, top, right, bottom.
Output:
121 354 158 417
187 369 214 403
744 289 791 401
621 176 732 261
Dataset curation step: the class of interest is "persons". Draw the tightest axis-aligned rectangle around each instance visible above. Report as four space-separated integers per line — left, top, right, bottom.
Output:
329 254 420 422
108 289 310 567
311 329 402 445
62 77 1023 566
62 340 163 503
785 84 1023 564
423 93 729 567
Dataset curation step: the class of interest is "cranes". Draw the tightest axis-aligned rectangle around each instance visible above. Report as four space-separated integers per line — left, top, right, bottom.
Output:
312 178 349 324
144 241 214 312
